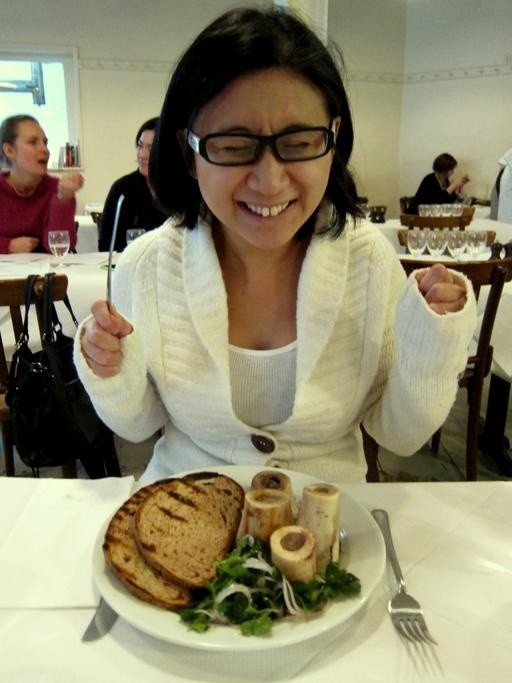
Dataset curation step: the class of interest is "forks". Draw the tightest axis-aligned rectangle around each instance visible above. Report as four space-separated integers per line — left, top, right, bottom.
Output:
371 509 437 646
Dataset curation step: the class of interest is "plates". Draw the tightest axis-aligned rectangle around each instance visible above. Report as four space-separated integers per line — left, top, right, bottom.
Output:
87 466 386 650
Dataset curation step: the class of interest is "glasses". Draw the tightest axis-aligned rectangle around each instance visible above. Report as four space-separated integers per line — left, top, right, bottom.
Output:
184 120 336 167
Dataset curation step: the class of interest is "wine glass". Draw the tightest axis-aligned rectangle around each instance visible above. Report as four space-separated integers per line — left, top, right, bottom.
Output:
48 231 70 270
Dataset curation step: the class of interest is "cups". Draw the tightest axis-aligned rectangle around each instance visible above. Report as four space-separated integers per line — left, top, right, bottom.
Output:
429 204 440 218
407 230 426 257
427 230 448 257
441 204 450 217
467 230 488 257
124 228 144 244
448 230 467 257
453 203 462 216
418 203 429 217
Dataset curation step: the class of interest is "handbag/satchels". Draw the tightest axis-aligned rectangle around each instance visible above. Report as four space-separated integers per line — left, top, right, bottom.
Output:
5 273 114 468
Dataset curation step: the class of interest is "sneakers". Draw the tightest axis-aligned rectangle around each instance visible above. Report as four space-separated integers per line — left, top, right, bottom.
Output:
479 446 512 480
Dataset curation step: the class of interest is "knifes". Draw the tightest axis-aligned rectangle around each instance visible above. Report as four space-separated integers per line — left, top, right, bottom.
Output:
81 598 121 642
108 195 126 309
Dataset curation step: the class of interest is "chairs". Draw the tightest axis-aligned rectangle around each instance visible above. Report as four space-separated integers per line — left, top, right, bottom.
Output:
400 215 472 230
399 196 412 212
0 274 81 477
474 291 512 479
361 257 511 482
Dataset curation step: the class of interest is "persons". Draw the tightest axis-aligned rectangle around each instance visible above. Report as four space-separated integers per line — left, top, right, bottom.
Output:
72 4 480 484
407 153 470 213
0 114 86 255
98 116 170 253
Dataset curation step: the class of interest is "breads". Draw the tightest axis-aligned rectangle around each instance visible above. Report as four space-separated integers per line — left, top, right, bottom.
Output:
102 473 244 610
